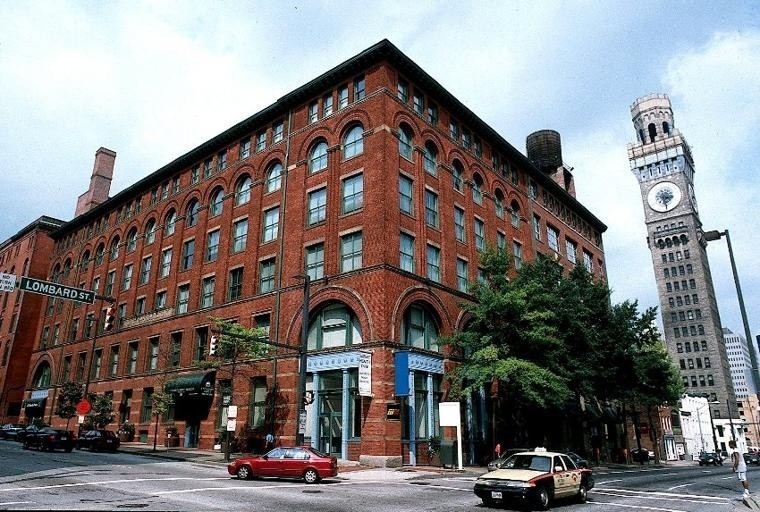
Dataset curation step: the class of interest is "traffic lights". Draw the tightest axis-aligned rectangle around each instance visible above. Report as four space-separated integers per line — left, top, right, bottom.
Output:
102 307 116 332
209 334 219 358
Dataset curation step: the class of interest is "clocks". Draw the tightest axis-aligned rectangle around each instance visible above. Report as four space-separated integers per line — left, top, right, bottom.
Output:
647 180 683 213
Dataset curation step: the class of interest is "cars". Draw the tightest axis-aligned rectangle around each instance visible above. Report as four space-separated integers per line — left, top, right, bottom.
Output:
0 421 122 454
696 449 760 466
225 444 340 485
629 445 655 462
476 445 595 510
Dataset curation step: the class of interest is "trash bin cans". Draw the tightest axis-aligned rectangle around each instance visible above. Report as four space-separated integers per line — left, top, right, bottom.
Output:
165 428 179 447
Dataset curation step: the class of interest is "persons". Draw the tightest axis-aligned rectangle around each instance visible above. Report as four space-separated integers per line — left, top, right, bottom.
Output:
495 441 502 459
265 430 274 452
729 440 751 499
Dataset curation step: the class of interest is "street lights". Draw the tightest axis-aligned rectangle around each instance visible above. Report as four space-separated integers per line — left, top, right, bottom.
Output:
702 224 759 405
748 404 760 448
291 270 311 445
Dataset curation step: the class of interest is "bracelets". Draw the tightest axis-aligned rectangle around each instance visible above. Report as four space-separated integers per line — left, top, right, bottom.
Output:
266 445 268 445
734 464 738 467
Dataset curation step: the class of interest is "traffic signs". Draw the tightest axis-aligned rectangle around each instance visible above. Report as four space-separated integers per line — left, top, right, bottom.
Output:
0 273 18 292
17 274 96 306
